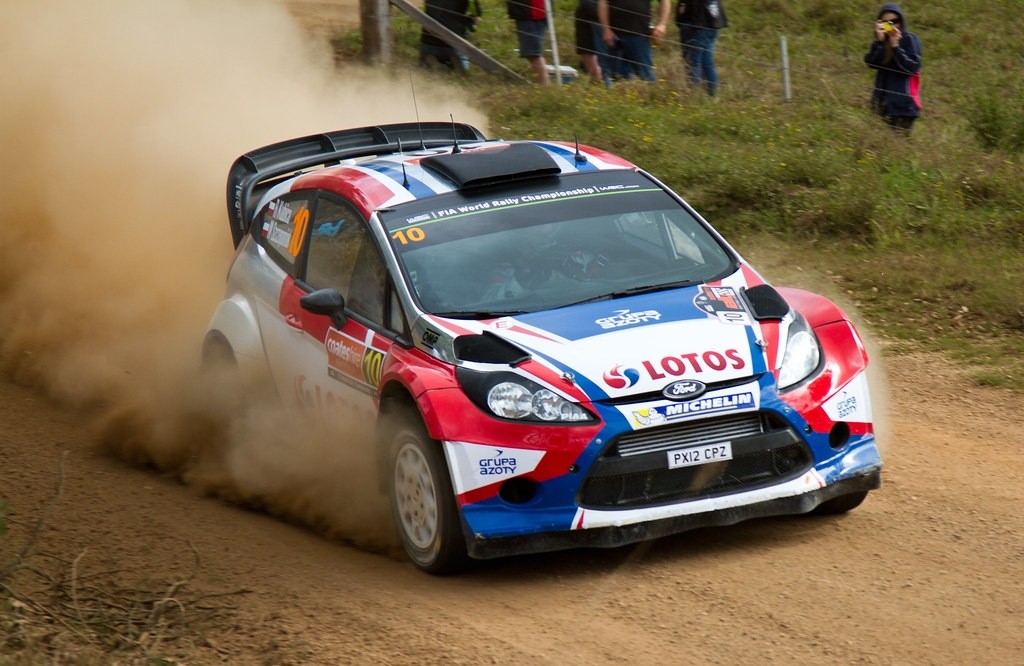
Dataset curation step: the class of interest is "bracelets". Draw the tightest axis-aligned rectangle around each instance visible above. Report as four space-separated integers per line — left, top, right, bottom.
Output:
657 24 666 34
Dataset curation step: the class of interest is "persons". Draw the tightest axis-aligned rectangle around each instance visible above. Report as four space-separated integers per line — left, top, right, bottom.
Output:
506 0 550 86
480 223 610 303
675 0 728 97
419 0 482 72
864 2 923 138
575 0 671 83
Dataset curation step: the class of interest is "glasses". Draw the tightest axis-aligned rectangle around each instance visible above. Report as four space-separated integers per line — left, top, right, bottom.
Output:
883 19 900 24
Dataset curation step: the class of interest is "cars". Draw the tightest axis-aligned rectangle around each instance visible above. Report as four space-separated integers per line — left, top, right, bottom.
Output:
197 121 881 576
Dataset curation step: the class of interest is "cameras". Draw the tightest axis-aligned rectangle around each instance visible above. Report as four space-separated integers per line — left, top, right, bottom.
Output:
880 22 894 32
